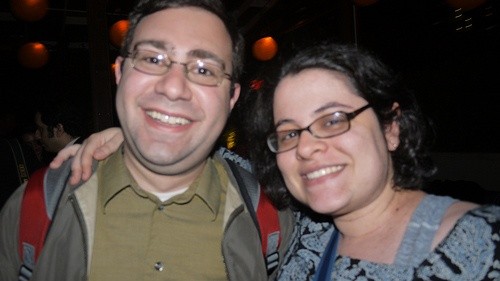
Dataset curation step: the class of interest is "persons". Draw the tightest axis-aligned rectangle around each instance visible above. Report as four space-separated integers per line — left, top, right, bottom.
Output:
0 0 297 281
49 43 500 281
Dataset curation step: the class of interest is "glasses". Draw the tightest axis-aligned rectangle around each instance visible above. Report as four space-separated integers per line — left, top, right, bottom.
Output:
266 98 380 154
122 49 234 85
